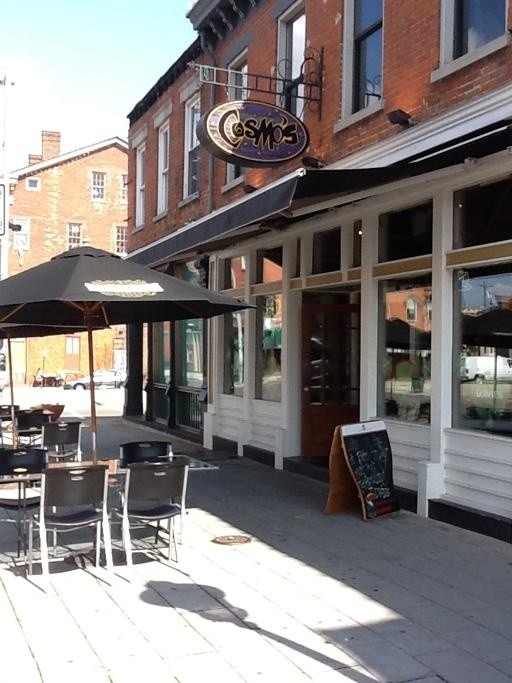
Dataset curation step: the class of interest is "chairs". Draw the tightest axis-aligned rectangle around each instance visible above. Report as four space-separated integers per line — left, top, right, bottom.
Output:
0 404 190 594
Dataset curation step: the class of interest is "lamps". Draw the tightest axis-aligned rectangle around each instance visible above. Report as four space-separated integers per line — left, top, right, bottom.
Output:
387 110 411 126
244 185 256 193
301 157 319 167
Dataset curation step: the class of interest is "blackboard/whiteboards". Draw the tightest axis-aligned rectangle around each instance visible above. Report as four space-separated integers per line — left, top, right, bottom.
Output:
340 419 401 522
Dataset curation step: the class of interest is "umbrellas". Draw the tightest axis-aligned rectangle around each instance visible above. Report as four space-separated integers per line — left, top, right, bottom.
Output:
459 306 512 410
385 316 429 400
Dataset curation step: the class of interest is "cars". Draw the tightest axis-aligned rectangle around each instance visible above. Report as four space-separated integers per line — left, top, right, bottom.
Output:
64 368 126 390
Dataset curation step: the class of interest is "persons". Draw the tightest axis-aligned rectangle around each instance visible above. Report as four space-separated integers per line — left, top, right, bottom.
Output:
261 317 281 375
36 367 44 386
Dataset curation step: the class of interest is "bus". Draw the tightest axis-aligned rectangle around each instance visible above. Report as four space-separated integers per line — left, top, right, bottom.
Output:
461 344 512 384
